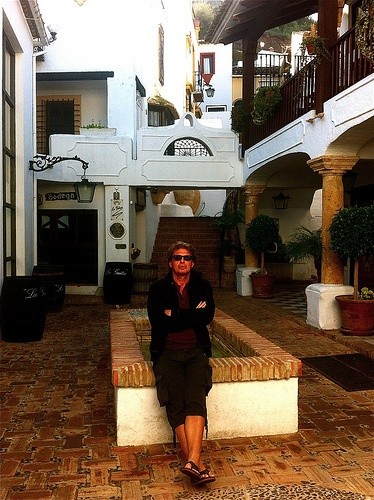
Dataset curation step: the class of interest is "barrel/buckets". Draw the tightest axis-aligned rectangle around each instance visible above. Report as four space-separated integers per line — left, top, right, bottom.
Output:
132 263 158 304
32 265 65 312
0 276 49 343
103 262 133 305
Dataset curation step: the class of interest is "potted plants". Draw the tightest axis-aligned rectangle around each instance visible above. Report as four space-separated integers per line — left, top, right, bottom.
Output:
79 117 117 136
302 26 330 62
329 205 374 336
250 83 281 116
244 215 280 299
284 226 322 283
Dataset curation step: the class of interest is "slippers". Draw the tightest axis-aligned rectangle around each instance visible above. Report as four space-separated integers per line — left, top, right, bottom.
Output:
191 466 216 486
180 461 200 479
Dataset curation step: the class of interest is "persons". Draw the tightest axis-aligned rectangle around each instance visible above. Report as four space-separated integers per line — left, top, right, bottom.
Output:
147 242 217 485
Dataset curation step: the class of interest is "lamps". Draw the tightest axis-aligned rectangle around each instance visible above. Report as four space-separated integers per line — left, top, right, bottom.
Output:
114 192 119 200
203 81 215 97
29 155 98 205
271 189 291 211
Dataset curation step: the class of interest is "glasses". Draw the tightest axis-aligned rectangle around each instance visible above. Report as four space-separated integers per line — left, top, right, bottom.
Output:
171 255 192 261
